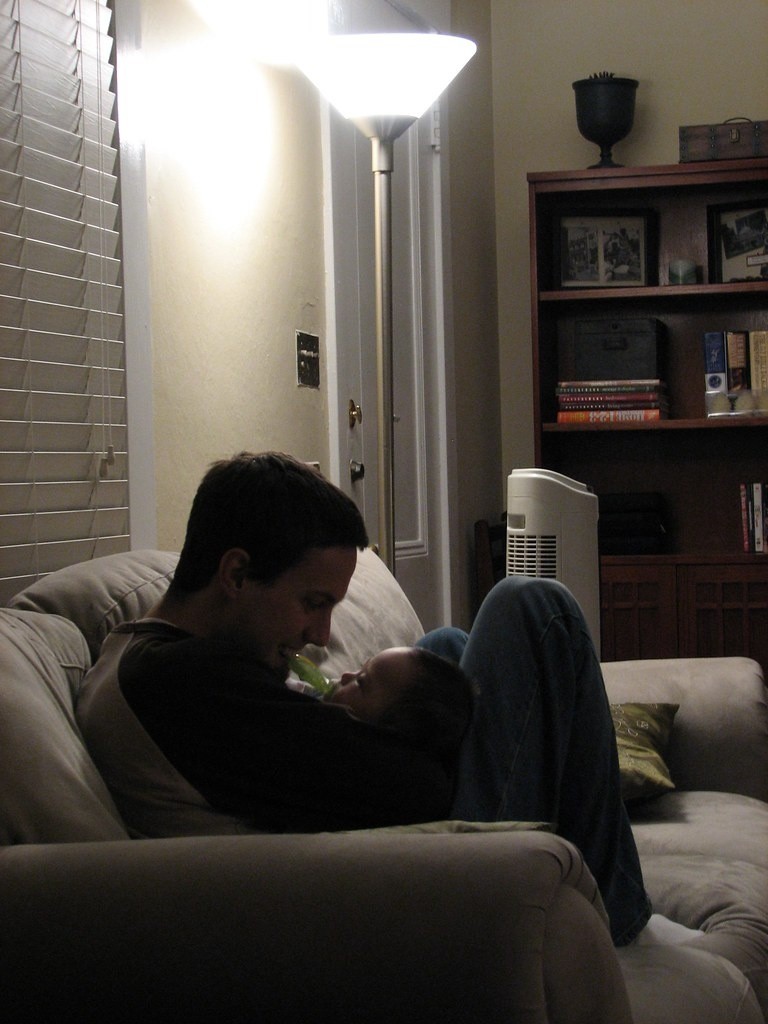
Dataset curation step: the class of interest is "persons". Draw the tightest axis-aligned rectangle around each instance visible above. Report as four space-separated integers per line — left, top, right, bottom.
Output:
73 450 707 952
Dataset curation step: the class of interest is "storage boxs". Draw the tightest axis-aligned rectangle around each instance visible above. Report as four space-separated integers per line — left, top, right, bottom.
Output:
574 318 669 380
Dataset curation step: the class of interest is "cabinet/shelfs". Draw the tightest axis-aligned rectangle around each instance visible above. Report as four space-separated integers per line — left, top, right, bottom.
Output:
527 157 768 677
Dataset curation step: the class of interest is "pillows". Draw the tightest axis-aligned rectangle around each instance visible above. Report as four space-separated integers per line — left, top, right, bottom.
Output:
284 547 427 683
354 820 554 833
610 702 680 804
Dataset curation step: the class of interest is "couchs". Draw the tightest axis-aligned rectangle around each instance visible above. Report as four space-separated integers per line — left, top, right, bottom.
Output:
0 551 768 1024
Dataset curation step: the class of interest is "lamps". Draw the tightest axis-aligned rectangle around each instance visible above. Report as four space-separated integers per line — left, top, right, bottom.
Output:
284 34 477 584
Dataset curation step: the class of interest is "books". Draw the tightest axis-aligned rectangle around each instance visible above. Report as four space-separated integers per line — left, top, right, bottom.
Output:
555 330 768 422
739 482 768 554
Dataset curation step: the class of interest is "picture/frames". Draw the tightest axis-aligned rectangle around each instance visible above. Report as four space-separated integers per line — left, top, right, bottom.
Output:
551 207 654 289
706 197 768 284
